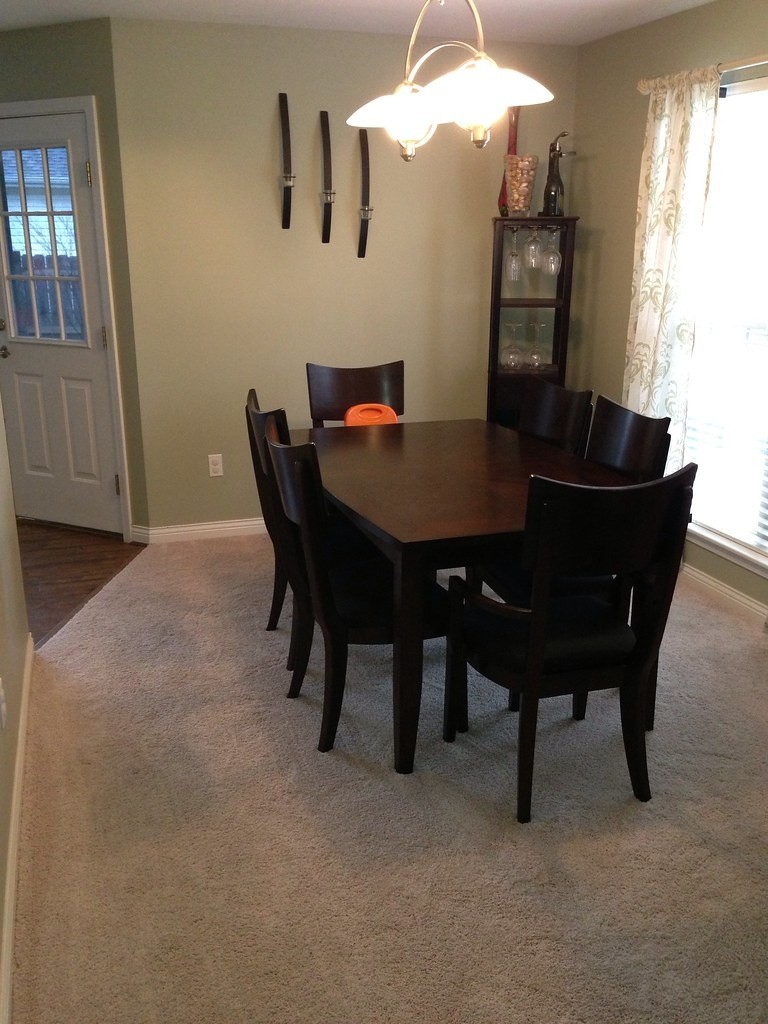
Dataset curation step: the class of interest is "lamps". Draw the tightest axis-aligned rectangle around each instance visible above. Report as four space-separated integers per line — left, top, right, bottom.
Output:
345 0 555 163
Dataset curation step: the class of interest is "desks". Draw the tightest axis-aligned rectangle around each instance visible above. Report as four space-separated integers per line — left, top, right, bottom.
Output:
288 418 644 775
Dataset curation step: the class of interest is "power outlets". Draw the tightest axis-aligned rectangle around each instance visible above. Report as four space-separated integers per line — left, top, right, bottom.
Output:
208 454 224 477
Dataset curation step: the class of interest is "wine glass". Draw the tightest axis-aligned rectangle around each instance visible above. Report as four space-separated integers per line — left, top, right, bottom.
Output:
501 324 524 370
505 225 562 282
526 324 548 370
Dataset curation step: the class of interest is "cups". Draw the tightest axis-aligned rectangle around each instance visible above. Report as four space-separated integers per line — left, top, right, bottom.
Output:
503 155 539 216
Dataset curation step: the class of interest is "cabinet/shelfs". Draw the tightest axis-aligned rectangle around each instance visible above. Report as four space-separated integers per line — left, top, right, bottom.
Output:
486 216 580 424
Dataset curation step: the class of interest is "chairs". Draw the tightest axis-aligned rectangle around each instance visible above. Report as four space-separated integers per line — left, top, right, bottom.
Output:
262 434 471 752
240 386 367 675
466 375 677 609
445 462 701 823
304 360 404 429
